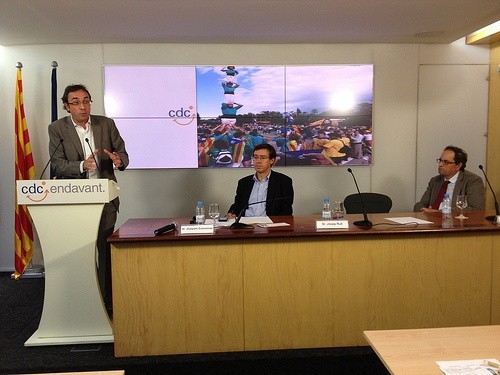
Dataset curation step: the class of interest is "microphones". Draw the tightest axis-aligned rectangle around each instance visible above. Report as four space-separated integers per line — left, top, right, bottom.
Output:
229 196 293 230
39 137 64 180
154 221 180 235
85 138 105 179
345 168 375 229
479 165 500 221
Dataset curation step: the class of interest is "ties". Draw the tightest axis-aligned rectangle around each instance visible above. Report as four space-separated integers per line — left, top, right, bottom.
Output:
432 181 451 210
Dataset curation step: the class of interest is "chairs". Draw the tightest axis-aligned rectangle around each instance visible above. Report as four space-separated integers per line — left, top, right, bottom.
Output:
343 192 392 214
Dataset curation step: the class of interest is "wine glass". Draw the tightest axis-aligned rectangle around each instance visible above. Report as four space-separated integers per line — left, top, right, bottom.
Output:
209 204 220 229
332 201 347 221
454 195 469 220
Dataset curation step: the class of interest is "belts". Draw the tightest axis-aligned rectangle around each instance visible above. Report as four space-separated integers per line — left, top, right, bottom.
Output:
353 143 362 144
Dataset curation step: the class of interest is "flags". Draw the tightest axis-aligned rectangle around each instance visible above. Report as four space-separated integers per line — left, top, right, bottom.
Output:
50 68 62 179
13 67 36 281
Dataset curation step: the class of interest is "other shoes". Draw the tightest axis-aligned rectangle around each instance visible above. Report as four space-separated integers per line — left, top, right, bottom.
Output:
105 291 112 311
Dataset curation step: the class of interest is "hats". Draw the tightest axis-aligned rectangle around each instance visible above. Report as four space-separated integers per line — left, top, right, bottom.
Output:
318 130 326 135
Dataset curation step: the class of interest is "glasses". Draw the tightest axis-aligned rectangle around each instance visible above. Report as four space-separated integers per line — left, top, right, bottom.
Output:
253 156 270 160
68 99 93 105
436 159 456 165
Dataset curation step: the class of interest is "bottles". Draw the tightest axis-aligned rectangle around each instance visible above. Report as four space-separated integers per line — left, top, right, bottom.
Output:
442 194 452 218
322 197 331 221
196 201 205 225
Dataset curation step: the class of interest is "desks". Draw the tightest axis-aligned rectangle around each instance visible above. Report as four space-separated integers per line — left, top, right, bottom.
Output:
107 212 500 357
363 325 500 375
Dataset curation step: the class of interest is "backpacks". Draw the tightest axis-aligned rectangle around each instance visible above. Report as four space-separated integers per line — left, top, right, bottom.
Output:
332 140 351 153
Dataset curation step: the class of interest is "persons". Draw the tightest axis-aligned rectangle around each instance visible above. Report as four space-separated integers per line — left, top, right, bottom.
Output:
225 143 294 219
196 66 373 166
48 86 129 314
413 146 486 213
